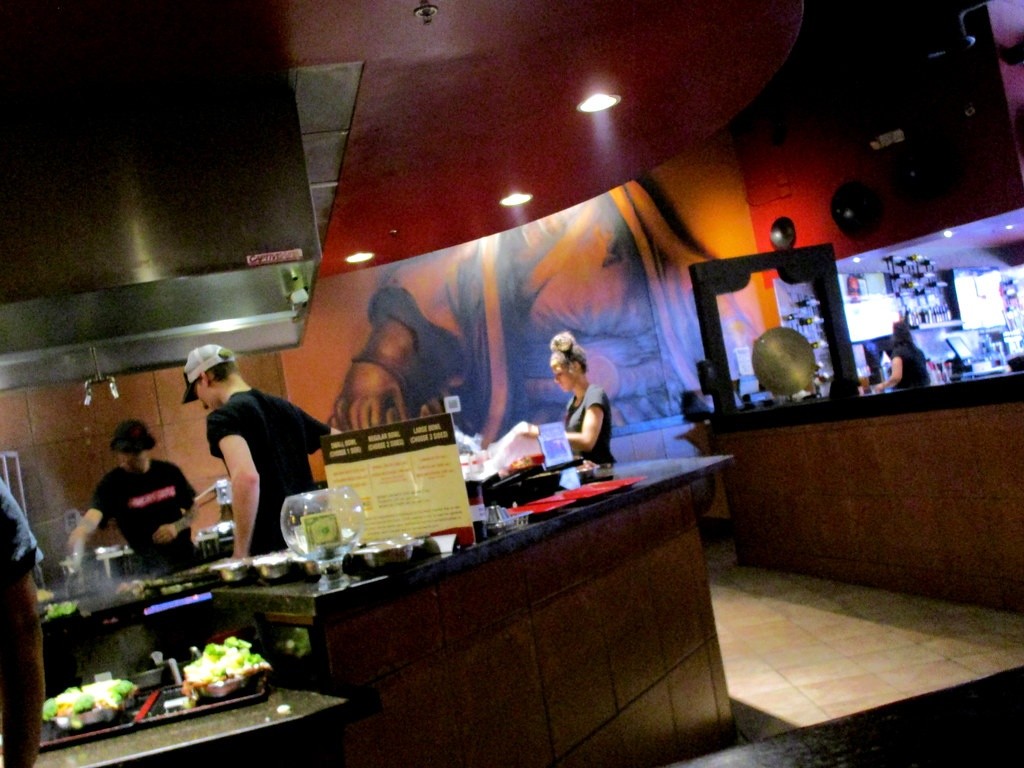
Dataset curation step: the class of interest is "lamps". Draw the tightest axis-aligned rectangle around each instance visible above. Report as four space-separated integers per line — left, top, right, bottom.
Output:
81 346 119 405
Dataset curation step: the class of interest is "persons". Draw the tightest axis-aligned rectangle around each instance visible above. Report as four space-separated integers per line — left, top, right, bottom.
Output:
179 343 342 561
0 473 45 768
873 321 931 392
60 419 204 577
515 332 617 466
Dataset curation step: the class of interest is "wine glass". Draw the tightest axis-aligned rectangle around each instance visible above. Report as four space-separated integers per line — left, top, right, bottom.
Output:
281 487 364 592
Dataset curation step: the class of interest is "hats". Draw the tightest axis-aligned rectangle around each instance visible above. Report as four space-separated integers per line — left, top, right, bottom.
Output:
182 345 235 404
111 420 157 452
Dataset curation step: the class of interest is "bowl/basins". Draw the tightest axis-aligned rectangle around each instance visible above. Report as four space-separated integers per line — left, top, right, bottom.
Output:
199 676 257 700
220 540 414 586
54 707 115 731
